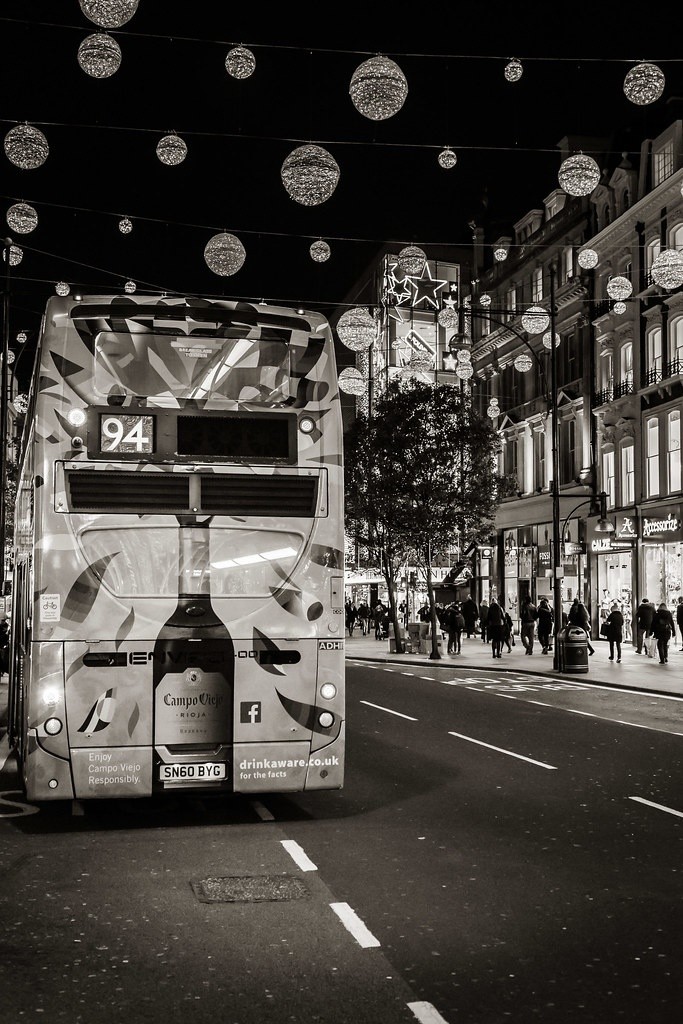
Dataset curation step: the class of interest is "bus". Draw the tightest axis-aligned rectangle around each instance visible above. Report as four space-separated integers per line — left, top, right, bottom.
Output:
9 292 347 812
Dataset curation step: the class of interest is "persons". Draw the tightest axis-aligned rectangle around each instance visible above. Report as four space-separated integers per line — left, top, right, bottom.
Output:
600 603 625 663
633 598 656 656
417 593 595 657
676 596 683 652
648 603 676 664
345 600 408 641
0 623 11 678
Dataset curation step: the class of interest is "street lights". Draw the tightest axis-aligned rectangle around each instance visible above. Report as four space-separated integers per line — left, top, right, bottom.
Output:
446 262 613 671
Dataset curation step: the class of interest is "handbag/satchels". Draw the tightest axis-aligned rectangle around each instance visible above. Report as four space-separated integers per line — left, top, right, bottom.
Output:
600 622 610 636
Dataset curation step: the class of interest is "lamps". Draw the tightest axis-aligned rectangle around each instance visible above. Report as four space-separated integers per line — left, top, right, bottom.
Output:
0 0 683 418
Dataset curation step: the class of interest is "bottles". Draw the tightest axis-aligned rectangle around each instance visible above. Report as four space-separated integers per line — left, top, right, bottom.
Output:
151 522 235 796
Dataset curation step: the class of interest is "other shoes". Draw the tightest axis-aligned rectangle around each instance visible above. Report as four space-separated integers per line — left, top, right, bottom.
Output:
542 646 553 655
609 657 613 660
526 645 533 655
448 649 461 655
659 659 668 664
616 659 621 663
679 648 683 651
635 650 641 654
493 649 512 658
589 650 595 656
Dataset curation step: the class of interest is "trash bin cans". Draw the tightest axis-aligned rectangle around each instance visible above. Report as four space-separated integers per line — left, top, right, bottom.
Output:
557 626 589 674
408 622 429 653
